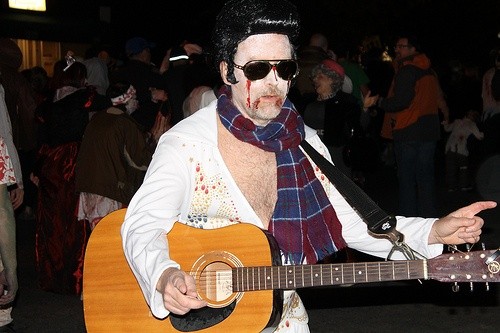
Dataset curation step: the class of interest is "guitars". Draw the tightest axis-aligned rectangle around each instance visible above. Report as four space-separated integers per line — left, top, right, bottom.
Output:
83 208 500 333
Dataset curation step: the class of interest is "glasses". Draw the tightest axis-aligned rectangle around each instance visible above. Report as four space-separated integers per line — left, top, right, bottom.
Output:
232 59 300 81
394 44 410 49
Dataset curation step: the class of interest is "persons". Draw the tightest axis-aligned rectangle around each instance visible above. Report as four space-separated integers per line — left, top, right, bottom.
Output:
121 0 497 333
0 36 500 326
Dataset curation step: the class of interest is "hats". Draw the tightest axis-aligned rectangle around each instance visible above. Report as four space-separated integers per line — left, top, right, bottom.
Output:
323 58 346 79
